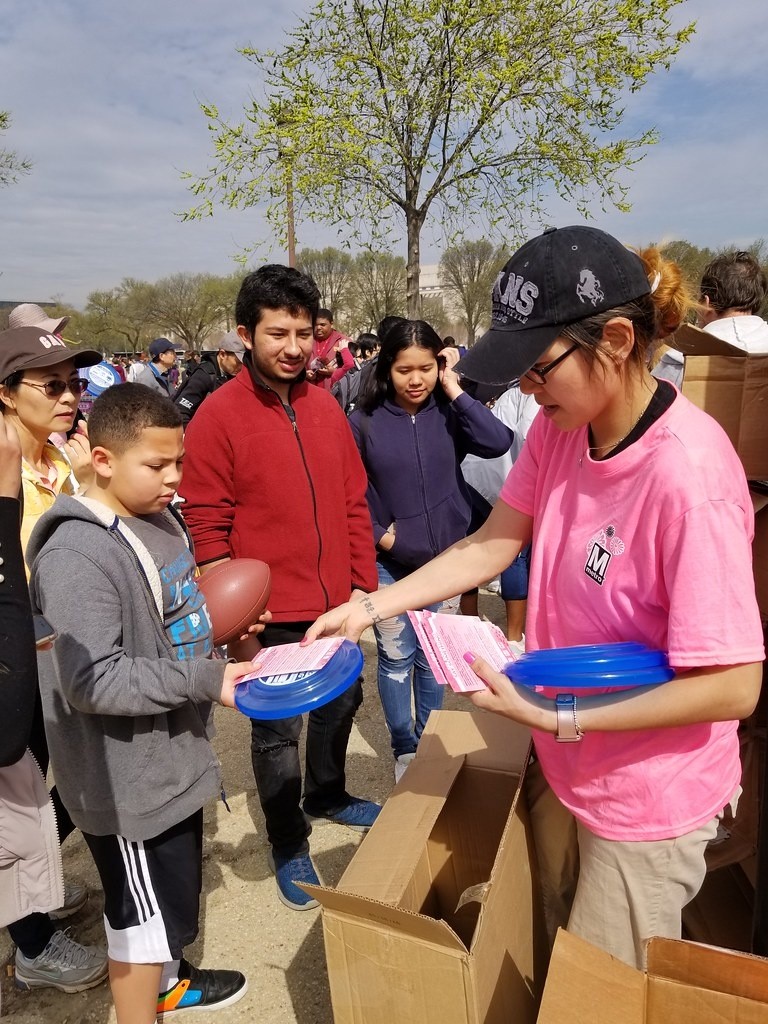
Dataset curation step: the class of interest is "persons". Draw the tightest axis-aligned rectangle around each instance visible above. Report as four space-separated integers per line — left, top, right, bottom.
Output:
0 327 109 993
698 251 768 353
306 308 405 411
460 386 541 639
348 320 514 784
24 382 272 1024
444 336 466 357
111 329 248 429
180 264 384 909
300 226 768 974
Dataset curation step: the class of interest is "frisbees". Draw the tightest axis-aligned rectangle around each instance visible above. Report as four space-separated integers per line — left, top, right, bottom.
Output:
235 638 365 720
502 640 676 686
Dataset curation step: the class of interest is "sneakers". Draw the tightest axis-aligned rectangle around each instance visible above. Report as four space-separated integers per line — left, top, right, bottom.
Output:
15 928 109 994
155 956 250 1018
44 886 87 920
326 797 383 830
272 847 323 909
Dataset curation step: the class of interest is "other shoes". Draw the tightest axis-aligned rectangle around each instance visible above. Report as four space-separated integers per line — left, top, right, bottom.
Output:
508 633 527 658
393 754 417 784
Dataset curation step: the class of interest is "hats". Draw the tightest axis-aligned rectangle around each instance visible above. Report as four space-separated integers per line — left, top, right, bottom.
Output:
8 303 70 336
149 338 182 357
218 331 245 363
451 225 652 387
0 327 102 382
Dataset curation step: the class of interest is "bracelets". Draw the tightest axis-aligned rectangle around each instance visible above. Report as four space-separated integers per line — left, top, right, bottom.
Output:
574 696 584 736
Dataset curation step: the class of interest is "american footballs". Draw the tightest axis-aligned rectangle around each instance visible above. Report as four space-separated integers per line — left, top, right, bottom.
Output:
193 558 272 647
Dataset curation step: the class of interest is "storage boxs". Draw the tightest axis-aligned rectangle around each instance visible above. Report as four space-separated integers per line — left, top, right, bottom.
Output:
290 709 768 1024
663 323 768 628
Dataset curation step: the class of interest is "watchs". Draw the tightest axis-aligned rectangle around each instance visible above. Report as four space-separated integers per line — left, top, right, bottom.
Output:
555 692 583 744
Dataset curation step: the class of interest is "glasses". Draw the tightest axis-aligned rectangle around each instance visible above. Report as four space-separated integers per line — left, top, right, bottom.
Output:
524 334 589 384
17 377 92 396
